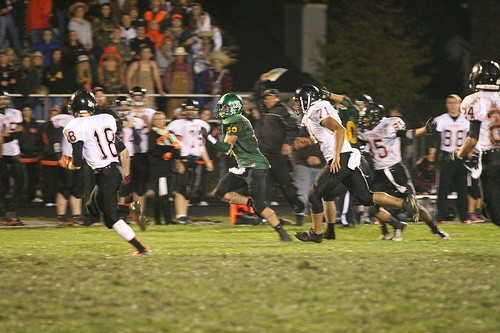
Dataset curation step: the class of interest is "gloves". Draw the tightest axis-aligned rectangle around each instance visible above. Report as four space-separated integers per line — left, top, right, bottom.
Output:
424 117 437 133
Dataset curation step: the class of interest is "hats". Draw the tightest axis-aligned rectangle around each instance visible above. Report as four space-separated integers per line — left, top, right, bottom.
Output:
69 2 88 14
171 47 188 56
261 88 280 97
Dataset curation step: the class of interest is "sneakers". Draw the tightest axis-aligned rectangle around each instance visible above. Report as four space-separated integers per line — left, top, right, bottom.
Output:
405 193 420 224
133 248 147 256
294 228 323 243
128 200 150 230
322 231 335 240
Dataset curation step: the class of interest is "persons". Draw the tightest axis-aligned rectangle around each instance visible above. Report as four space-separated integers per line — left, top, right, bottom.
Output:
449 58 500 227
163 45 196 94
126 44 166 96
50 88 153 257
42 47 68 94
198 93 293 243
0 0 500 256
200 48 240 94
425 93 473 226
251 67 305 227
148 111 181 226
167 98 215 206
0 89 26 226
95 45 125 94
294 83 421 243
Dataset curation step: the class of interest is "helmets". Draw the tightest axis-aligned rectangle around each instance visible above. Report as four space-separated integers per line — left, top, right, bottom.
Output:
181 98 200 110
67 89 97 117
292 84 320 110
129 86 146 98
360 103 383 130
216 93 244 119
468 59 500 91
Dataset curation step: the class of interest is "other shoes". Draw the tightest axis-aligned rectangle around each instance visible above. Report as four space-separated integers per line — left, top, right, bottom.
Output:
439 234 451 240
72 215 100 227
436 219 445 224
56 218 79 228
0 216 24 228
393 221 407 241
174 216 193 225
280 235 293 242
379 233 393 240
464 214 491 223
